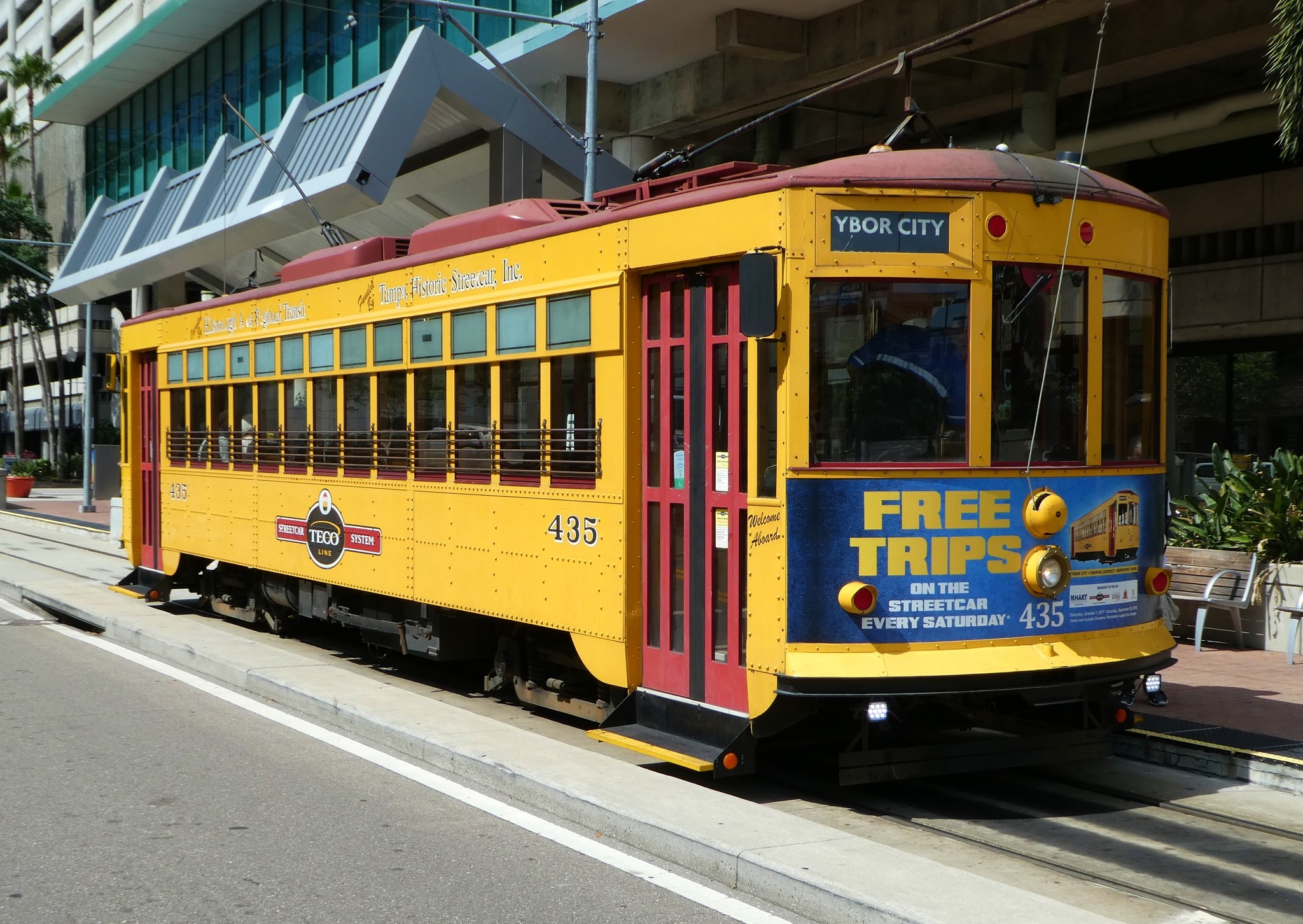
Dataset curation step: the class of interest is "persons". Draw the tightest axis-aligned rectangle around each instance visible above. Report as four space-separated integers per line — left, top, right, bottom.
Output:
197 414 261 463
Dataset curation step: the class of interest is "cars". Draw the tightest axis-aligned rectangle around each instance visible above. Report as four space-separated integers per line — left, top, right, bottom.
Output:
1194 463 1223 502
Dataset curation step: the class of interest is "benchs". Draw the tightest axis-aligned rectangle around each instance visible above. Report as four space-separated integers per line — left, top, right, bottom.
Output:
1164 546 1257 651
1274 588 1303 664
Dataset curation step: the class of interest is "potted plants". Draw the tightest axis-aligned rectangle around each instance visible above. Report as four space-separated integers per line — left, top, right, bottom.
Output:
6 460 37 498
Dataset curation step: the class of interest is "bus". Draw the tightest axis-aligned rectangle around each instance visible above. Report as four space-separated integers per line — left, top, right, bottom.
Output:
104 1 1180 781
